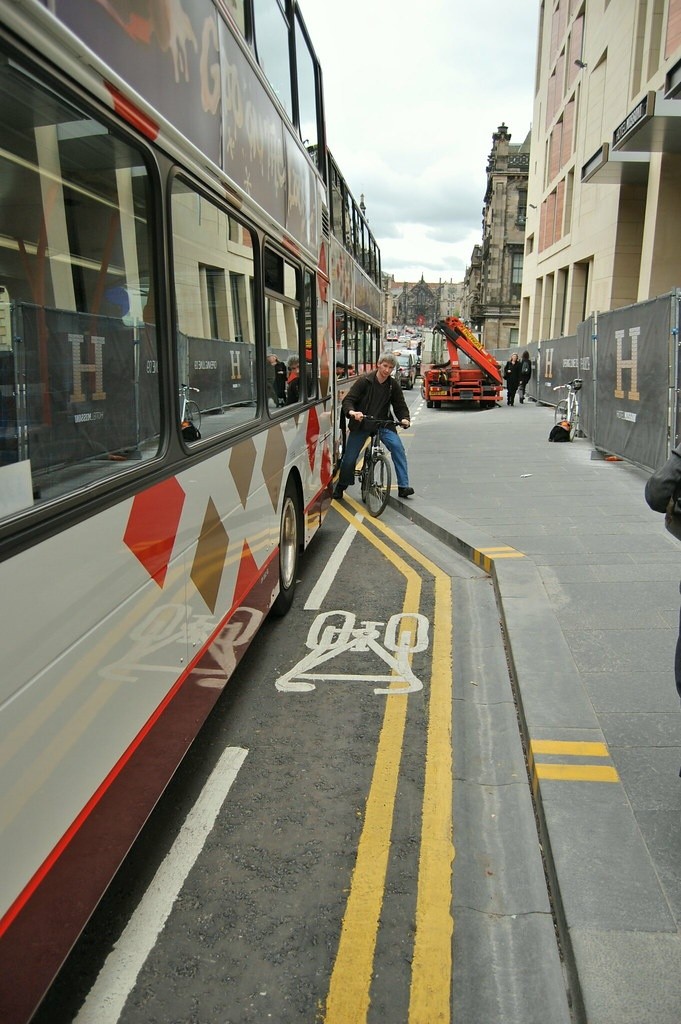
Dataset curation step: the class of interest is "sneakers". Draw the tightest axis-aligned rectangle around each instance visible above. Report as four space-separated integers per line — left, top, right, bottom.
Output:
398 487 414 498
333 482 348 498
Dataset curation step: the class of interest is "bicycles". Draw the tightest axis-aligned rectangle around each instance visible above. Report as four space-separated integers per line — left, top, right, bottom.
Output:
552 379 583 442
176 384 203 432
351 414 411 518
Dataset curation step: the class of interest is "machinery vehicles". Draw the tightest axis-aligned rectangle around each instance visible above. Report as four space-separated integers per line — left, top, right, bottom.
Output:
420 315 504 408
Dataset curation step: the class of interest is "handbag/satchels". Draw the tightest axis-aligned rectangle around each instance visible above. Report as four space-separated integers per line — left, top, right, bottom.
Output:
503 362 510 380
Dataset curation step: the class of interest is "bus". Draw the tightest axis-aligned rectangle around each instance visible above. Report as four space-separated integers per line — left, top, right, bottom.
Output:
0 0 389 1022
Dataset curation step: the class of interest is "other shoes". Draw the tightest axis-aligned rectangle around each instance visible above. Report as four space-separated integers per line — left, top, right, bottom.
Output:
520 397 525 404
511 401 514 406
507 399 510 405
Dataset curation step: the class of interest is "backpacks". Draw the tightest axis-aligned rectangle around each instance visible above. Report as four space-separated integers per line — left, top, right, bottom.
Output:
521 361 531 375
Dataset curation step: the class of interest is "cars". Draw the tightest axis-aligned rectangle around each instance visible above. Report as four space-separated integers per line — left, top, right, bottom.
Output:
389 349 421 390
387 328 425 349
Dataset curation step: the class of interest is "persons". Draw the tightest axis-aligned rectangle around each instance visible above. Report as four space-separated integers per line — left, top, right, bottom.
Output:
332 351 415 500
644 442 681 698
503 353 523 406
268 353 313 406
335 362 356 379
518 350 531 404
